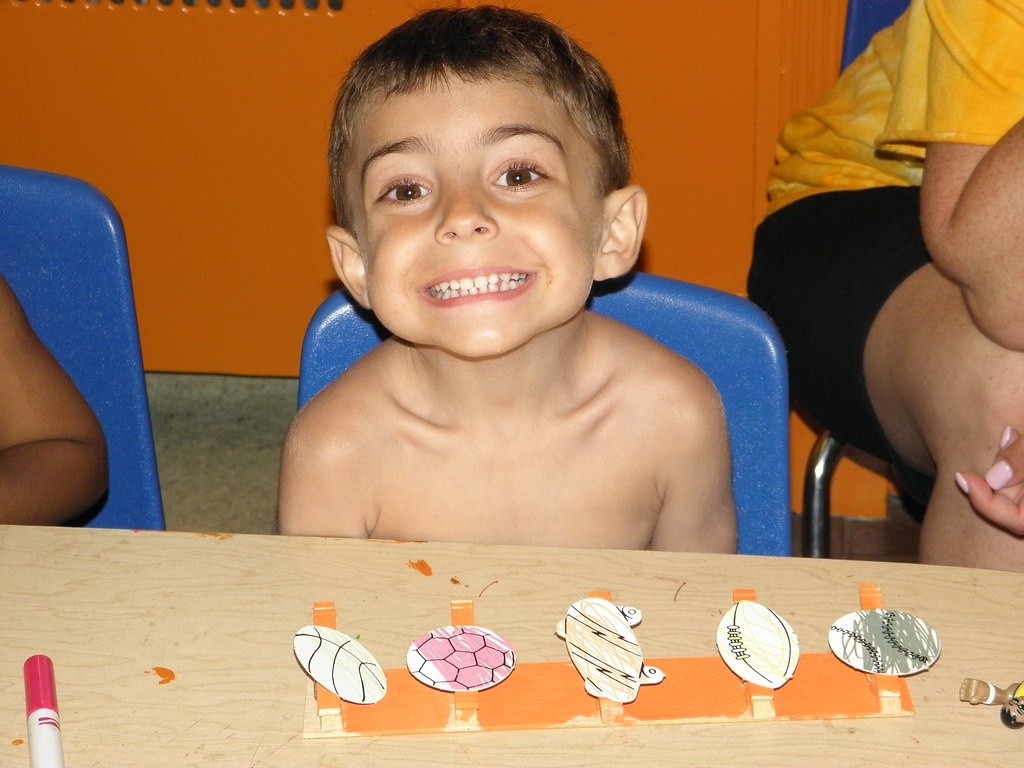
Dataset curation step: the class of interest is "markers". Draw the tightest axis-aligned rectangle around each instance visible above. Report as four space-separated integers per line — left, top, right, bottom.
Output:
24 652 64 768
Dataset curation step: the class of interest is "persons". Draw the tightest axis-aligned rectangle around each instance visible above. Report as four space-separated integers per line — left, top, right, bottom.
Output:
0 276 109 526
748 0 1024 574
276 5 741 553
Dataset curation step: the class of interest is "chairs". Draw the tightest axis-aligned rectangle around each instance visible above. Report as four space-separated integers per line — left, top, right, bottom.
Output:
0 168 165 531
805 0 932 559
299 270 793 555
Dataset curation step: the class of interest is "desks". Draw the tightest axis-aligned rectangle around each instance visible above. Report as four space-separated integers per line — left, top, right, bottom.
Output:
0 519 1024 768
